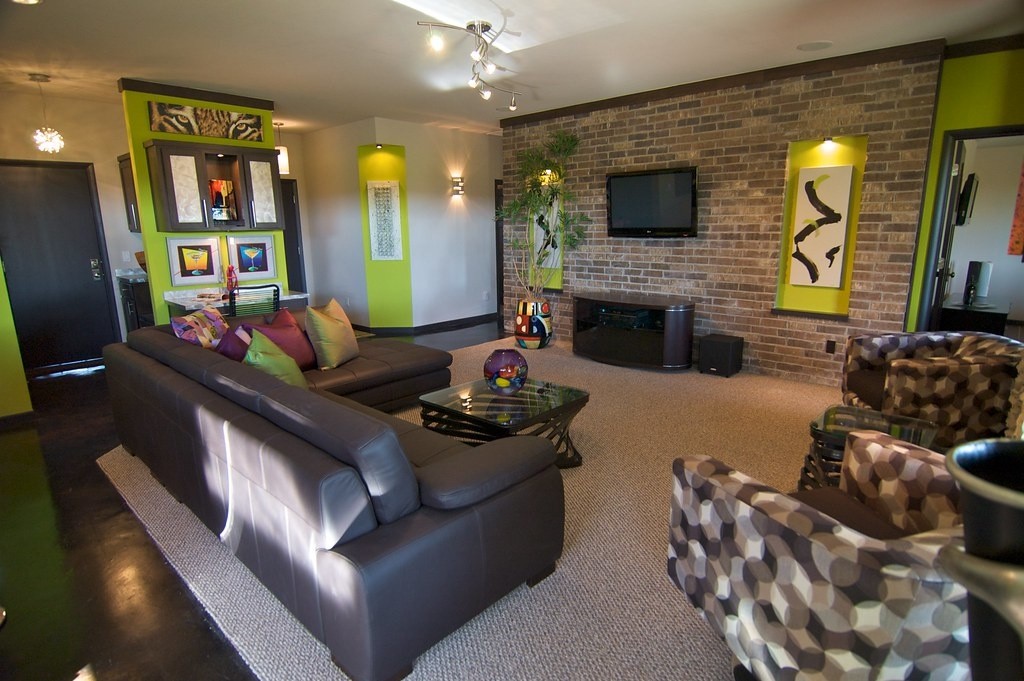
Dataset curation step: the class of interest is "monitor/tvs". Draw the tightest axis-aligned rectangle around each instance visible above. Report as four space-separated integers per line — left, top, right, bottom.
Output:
606 165 700 239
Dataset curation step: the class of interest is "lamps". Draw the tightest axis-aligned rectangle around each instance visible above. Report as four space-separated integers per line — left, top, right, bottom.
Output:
271 123 291 175
453 176 465 196
824 137 835 142
377 144 383 149
28 71 63 154
545 168 552 175
419 21 521 111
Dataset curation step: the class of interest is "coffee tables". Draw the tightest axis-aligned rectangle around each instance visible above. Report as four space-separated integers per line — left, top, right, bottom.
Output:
418 380 590 468
796 406 940 490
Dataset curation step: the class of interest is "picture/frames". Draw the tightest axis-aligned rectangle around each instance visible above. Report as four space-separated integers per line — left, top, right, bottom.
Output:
166 237 224 286
227 234 277 282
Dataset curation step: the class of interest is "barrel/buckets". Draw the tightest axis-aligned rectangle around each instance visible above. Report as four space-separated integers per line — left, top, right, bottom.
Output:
944 435 1024 681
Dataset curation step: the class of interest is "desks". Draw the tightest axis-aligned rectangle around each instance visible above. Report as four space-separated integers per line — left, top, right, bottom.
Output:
935 297 1010 333
577 293 695 371
163 284 310 320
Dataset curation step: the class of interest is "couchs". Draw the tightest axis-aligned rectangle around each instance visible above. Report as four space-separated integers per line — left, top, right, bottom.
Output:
670 429 973 681
105 307 566 680
841 329 1024 449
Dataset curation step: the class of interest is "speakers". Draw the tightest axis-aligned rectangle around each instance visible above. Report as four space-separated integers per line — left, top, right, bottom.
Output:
698 334 744 378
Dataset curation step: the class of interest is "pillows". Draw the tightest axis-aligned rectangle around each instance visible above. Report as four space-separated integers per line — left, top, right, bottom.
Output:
170 297 359 392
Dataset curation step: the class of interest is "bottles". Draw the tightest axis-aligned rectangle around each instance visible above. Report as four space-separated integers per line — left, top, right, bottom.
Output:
483 348 528 397
965 275 976 306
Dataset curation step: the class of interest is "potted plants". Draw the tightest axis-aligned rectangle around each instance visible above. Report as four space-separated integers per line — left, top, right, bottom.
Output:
495 133 591 347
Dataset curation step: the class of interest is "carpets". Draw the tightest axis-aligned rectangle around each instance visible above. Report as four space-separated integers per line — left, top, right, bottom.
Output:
93 332 864 681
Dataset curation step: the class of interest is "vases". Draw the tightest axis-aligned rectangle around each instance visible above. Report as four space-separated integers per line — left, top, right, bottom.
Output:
484 347 528 396
